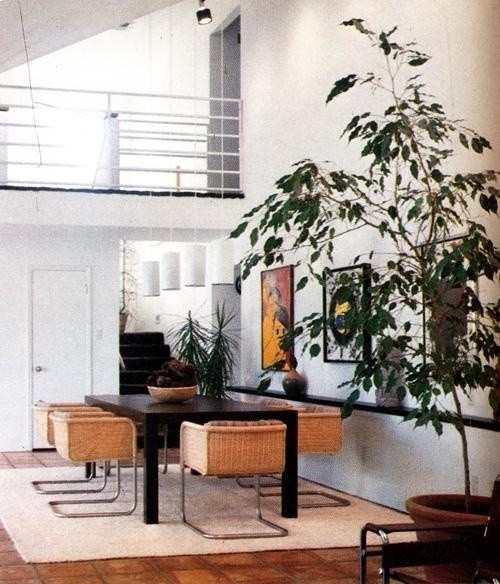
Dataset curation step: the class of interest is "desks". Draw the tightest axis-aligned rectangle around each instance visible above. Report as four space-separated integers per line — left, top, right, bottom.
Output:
85 393 299 526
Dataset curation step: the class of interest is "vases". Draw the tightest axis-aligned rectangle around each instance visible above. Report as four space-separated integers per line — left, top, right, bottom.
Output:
282 366 307 396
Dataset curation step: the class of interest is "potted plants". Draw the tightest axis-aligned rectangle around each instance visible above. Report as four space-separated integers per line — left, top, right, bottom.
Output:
218 17 499 582
119 238 141 334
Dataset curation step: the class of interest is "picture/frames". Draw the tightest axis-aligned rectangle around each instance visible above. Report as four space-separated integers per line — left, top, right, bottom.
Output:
322 263 371 365
260 263 294 373
421 235 480 367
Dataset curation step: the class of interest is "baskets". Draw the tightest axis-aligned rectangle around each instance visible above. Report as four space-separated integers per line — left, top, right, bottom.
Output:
148 385 198 403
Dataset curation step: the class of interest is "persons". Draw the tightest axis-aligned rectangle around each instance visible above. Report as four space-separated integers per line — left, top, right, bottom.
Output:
267 309 290 371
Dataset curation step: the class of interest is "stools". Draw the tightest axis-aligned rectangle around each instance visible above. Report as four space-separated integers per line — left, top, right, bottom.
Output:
103 419 168 478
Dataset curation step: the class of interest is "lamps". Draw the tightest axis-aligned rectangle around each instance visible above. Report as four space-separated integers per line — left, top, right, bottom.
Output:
138 0 236 298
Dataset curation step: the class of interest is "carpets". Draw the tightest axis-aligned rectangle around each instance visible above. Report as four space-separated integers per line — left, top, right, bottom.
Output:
0 463 417 564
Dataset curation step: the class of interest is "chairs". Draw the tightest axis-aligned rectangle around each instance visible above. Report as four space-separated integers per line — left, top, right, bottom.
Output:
254 404 350 509
32 402 109 495
179 420 289 539
218 400 294 489
356 475 500 583
48 411 139 518
